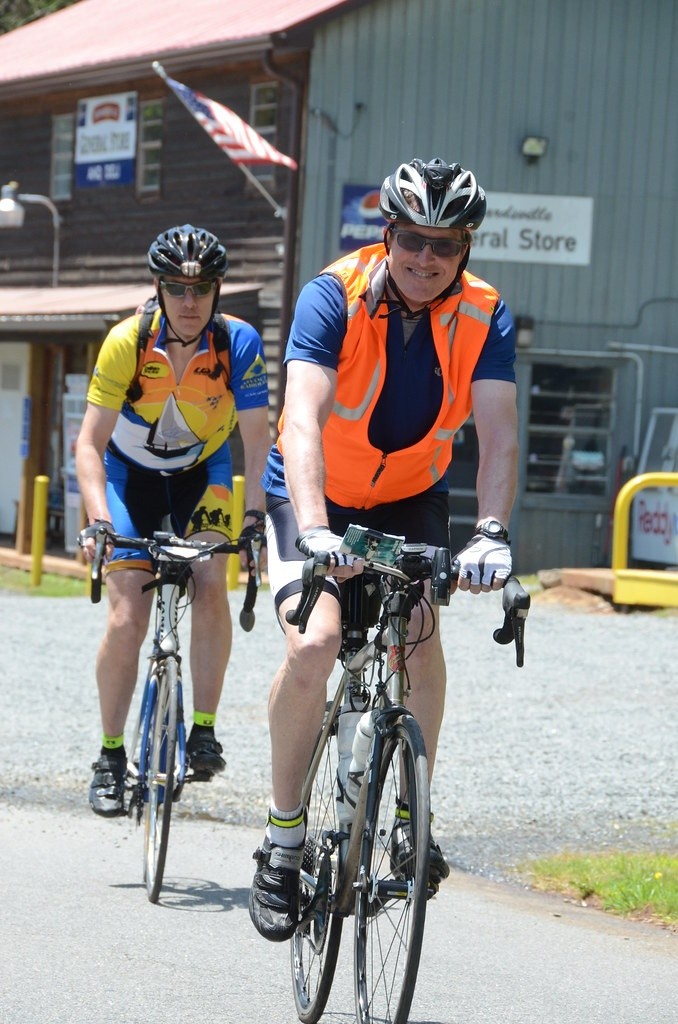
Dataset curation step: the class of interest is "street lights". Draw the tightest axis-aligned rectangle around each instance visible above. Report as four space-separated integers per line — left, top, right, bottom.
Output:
0 182 63 295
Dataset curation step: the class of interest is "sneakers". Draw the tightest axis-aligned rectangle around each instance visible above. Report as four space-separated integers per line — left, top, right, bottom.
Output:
248 836 307 942
89 753 128 816
390 822 449 882
186 733 226 774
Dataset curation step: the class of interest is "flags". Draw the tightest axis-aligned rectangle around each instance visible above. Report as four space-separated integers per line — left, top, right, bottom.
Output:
157 68 297 170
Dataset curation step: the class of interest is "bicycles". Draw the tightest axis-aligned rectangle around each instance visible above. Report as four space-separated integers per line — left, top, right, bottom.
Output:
78 516 277 905
284 524 531 1024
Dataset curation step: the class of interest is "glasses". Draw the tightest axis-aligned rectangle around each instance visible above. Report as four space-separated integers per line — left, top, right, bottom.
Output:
388 225 468 259
159 280 215 298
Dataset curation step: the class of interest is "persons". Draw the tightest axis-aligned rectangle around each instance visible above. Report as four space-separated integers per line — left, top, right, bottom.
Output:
247 156 520 943
76 224 271 817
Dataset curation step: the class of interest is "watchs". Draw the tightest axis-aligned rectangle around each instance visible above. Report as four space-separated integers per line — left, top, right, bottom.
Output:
475 519 511 546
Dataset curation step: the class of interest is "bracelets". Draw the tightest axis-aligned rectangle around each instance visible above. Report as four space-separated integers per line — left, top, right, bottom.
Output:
243 510 266 527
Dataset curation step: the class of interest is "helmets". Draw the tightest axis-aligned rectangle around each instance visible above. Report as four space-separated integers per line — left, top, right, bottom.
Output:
146 223 228 282
378 157 488 230
